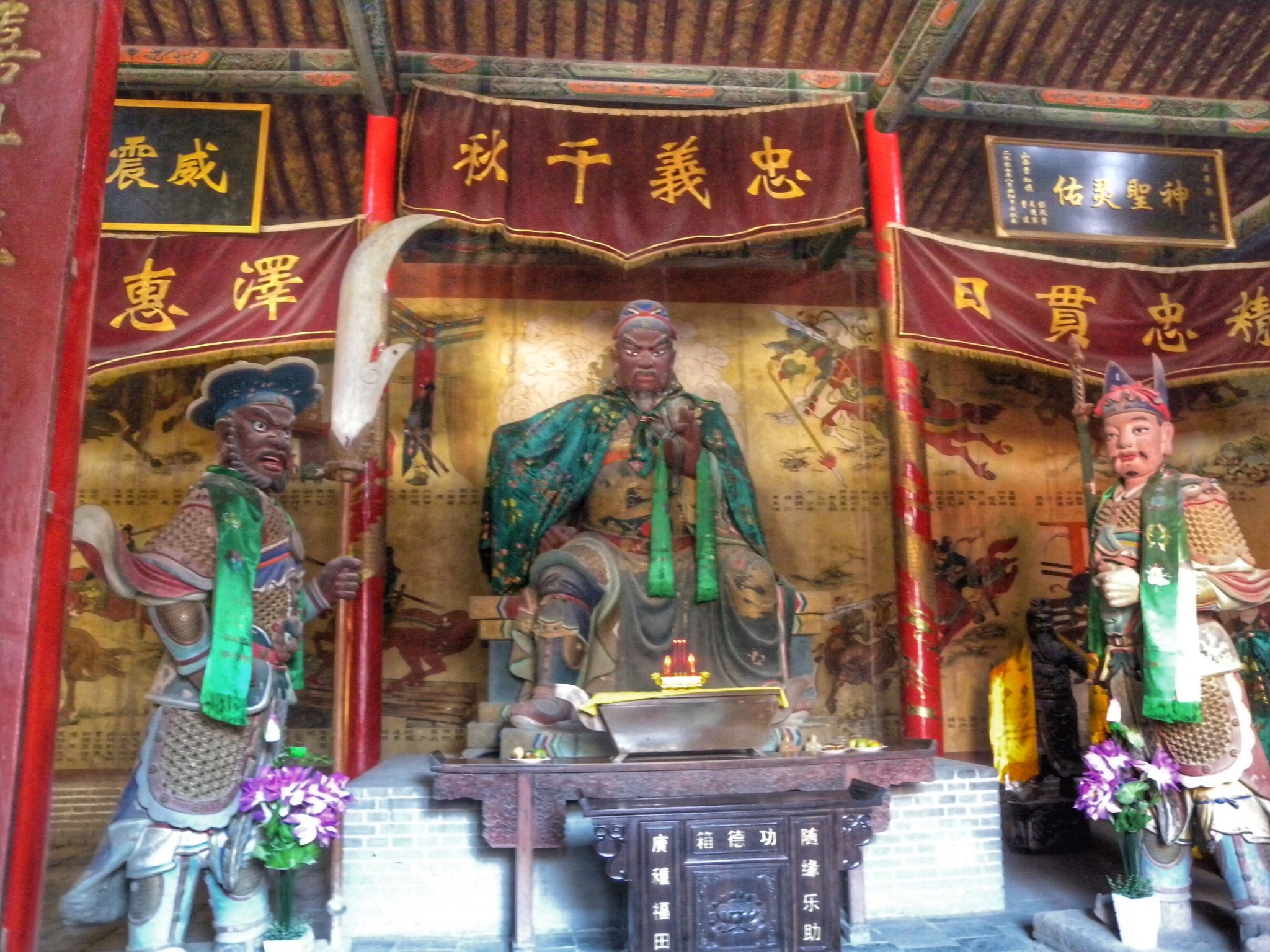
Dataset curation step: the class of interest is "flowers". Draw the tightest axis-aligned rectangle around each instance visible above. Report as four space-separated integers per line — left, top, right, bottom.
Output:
1079 728 1182 895
239 752 358 940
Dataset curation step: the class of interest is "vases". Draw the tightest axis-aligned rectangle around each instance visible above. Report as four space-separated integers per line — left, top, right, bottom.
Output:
1111 891 1161 949
263 925 315 951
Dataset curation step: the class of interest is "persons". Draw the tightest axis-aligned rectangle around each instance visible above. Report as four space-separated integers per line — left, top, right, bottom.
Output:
1087 359 1270 950
479 297 815 725
53 357 361 951
